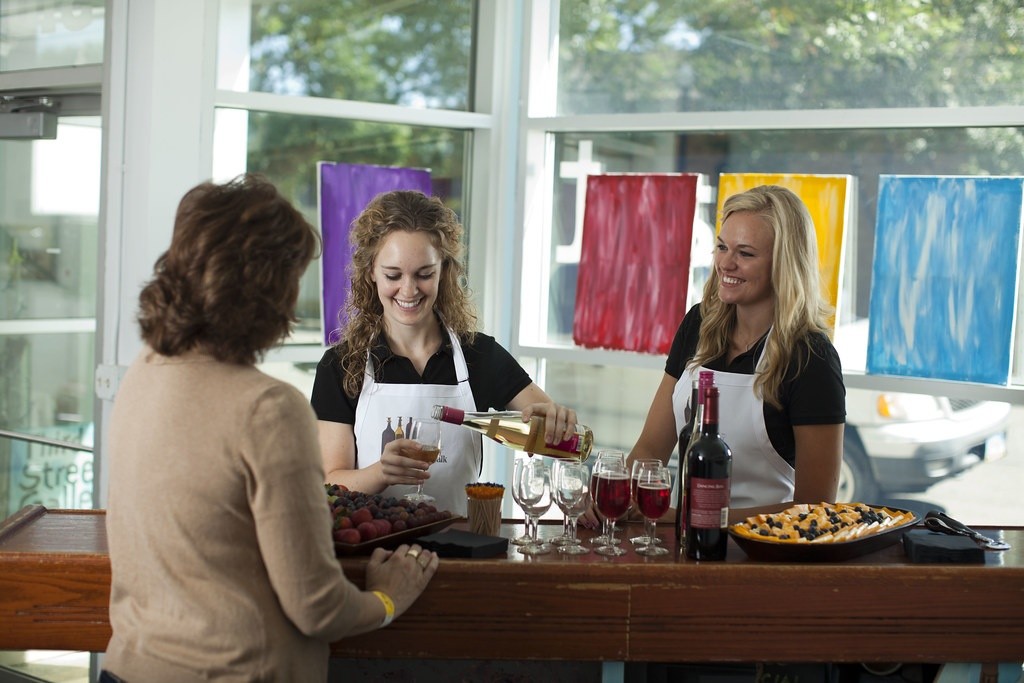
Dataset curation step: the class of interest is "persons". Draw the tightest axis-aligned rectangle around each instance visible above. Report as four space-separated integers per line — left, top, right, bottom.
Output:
95 173 440 683
579 185 847 530
310 191 576 520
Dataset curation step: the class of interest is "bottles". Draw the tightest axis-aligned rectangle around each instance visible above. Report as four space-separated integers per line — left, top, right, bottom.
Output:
380 416 416 457
430 406 593 464
675 371 732 560
684 395 691 422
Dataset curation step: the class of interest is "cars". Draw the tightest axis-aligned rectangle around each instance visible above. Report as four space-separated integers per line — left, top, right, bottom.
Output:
688 218 1011 503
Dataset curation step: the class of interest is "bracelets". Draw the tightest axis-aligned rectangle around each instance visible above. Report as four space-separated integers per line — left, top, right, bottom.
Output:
372 591 394 628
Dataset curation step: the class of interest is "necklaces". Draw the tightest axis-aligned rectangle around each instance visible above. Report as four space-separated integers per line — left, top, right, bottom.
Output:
744 330 762 352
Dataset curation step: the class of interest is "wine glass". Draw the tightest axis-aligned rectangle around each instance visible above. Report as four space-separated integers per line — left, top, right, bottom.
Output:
404 417 440 501
510 450 671 556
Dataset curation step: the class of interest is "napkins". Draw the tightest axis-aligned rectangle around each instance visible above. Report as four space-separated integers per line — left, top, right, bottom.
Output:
903 533 985 564
417 529 510 558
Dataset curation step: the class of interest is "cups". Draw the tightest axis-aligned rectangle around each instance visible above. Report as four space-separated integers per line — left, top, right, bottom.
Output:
466 496 503 538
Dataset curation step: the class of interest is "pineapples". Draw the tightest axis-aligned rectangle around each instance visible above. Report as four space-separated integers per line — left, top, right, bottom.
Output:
732 501 916 543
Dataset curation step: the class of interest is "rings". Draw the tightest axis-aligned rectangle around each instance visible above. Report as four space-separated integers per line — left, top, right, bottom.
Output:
406 550 419 558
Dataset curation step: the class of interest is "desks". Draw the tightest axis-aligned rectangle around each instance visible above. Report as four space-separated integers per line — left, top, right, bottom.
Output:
0 504 1024 683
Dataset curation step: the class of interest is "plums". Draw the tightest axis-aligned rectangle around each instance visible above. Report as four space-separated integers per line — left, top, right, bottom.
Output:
325 483 454 543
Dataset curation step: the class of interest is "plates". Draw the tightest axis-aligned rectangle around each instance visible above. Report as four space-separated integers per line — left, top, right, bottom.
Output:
728 504 923 560
